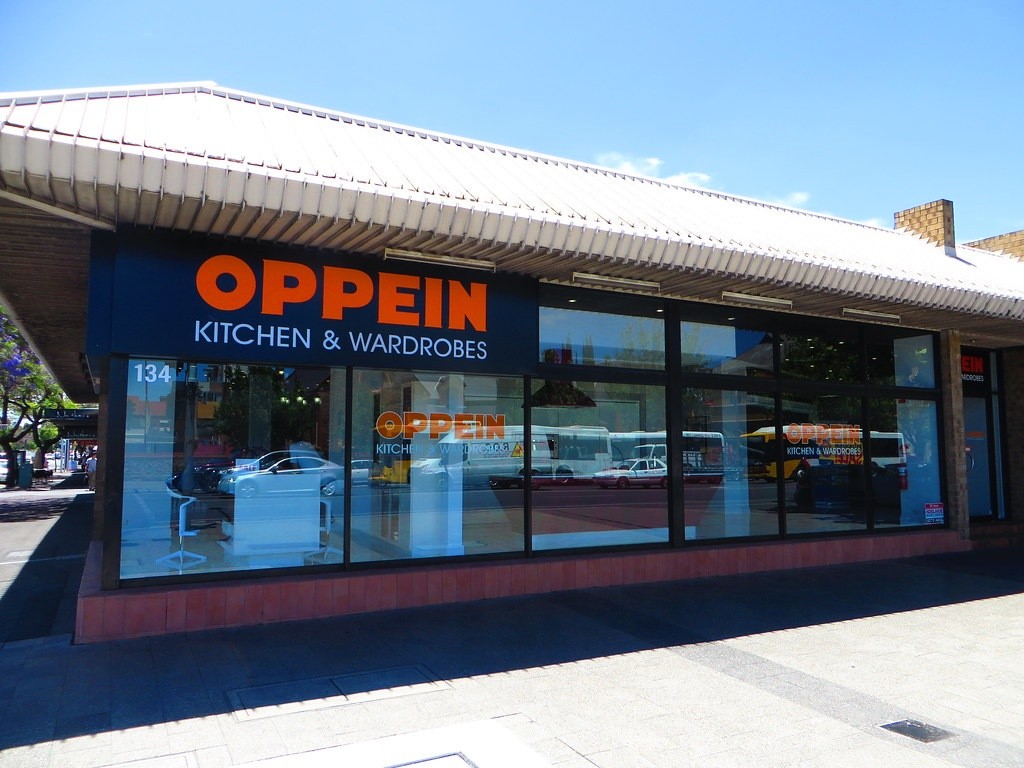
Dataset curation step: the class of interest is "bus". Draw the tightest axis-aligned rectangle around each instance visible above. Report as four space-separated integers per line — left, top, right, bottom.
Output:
368 419 484 488
408 425 908 489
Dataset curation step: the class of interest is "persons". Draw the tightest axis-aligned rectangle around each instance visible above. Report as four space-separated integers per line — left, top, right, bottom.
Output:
84 451 97 491
284 432 335 527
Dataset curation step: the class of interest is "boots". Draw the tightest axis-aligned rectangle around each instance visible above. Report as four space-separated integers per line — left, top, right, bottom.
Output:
208 523 231 541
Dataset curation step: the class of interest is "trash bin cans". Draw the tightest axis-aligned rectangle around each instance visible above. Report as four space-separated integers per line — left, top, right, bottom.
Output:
843 465 902 524
18 464 33 489
796 462 880 514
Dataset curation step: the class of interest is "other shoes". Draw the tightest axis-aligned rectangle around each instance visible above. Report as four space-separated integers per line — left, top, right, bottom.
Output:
320 518 336 526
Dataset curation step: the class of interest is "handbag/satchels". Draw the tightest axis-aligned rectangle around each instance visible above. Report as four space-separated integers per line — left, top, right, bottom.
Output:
84 473 89 482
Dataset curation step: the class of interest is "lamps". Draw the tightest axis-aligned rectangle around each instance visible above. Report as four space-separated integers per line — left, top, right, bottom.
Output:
842 307 901 324
721 291 792 310
572 272 660 293
382 248 496 275
0 184 117 233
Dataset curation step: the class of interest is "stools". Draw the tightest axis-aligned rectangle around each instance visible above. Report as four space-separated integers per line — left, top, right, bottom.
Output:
302 492 344 564
156 481 207 569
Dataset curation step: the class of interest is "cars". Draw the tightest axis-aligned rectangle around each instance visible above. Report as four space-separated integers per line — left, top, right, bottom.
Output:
347 459 374 486
164 449 355 497
0 451 63 483
